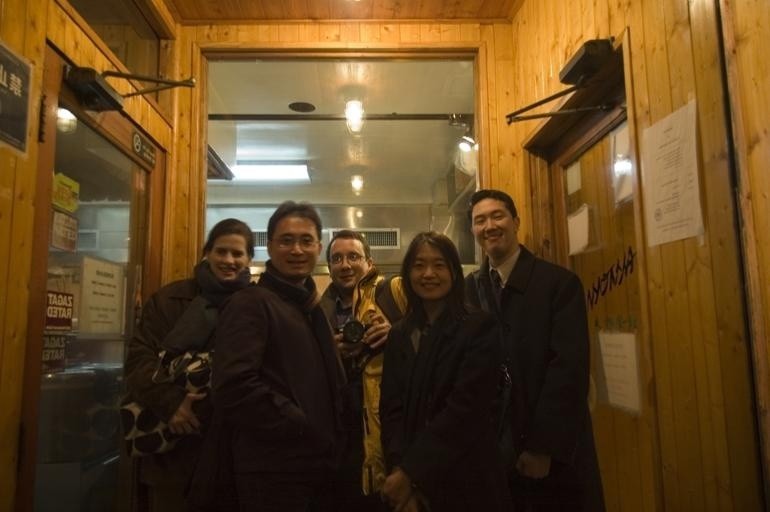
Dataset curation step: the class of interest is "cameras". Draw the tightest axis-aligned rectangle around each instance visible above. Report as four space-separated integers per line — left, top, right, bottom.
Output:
334 321 375 352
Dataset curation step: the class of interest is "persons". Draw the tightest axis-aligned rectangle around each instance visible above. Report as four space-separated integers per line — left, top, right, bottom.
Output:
462 187 607 511
209 199 351 512
121 218 255 511
379 229 506 512
318 229 411 510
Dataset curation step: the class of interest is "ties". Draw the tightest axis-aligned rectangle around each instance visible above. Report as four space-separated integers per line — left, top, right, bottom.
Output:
487 265 504 314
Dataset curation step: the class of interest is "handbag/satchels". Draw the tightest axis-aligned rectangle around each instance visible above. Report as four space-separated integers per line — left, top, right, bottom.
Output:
115 347 221 458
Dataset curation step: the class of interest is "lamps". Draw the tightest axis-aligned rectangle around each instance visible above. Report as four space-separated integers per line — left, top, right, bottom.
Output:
342 84 367 133
448 112 475 152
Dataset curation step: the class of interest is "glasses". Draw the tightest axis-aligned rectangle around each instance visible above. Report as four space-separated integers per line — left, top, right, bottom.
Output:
269 235 319 249
328 252 367 264
